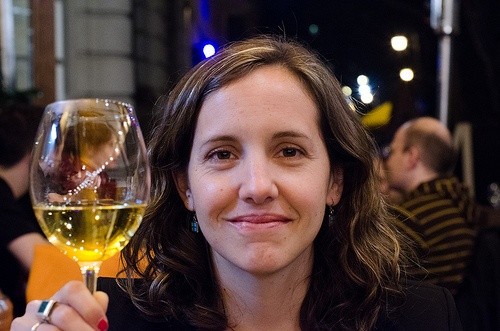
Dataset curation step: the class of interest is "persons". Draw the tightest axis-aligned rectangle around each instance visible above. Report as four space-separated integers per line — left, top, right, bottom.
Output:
9 34 464 331
382 116 483 297
0 91 63 301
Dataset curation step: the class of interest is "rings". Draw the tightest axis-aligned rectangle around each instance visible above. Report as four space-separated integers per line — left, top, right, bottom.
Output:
35 298 56 321
30 322 43 331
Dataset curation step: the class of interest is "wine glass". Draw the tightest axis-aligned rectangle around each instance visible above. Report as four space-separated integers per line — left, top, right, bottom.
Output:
28 98 151 296
